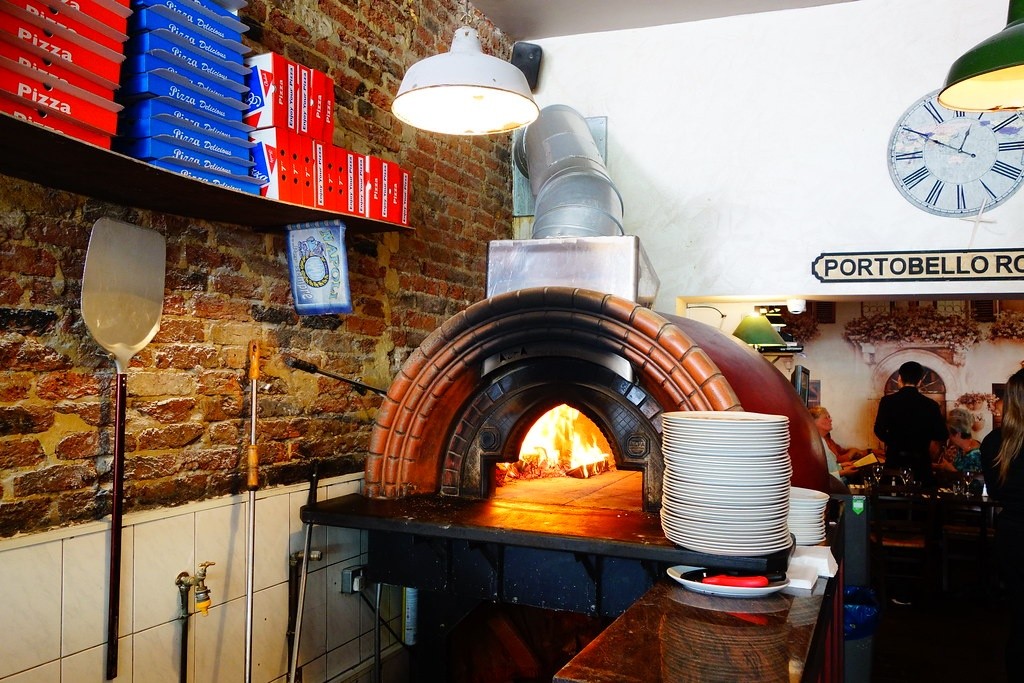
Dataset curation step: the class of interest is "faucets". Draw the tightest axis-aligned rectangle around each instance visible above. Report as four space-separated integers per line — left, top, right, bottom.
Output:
174 560 216 618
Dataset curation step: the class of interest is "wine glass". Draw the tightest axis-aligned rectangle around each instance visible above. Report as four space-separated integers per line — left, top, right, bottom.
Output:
898 467 912 497
964 470 973 497
873 465 883 496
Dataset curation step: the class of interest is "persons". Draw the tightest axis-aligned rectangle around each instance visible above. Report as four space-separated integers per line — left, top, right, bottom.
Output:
978 365 1024 683
808 362 983 610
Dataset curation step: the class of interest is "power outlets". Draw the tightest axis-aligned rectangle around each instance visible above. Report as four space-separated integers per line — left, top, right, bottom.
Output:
341 565 366 594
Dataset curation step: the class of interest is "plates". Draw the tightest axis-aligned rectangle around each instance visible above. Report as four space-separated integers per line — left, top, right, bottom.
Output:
660 410 829 555
667 565 789 598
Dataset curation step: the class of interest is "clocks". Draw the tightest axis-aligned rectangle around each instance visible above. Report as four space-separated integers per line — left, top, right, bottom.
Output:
887 88 1024 217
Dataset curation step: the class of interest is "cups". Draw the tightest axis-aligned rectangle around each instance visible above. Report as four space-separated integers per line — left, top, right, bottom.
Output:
864 477 873 494
953 480 964 495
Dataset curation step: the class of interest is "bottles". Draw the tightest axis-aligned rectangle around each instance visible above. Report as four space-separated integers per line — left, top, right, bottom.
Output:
890 477 897 496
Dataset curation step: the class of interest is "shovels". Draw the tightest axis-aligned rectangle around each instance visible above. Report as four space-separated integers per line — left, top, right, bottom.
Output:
80 217 166 681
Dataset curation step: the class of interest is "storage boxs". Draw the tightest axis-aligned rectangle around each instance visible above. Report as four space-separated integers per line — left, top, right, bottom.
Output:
0 0 410 226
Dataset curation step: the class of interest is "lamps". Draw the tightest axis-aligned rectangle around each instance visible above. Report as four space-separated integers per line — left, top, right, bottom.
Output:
938 0 1024 113
391 0 540 135
733 315 786 351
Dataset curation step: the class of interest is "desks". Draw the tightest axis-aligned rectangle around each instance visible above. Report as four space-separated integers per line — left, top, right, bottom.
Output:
552 558 843 683
